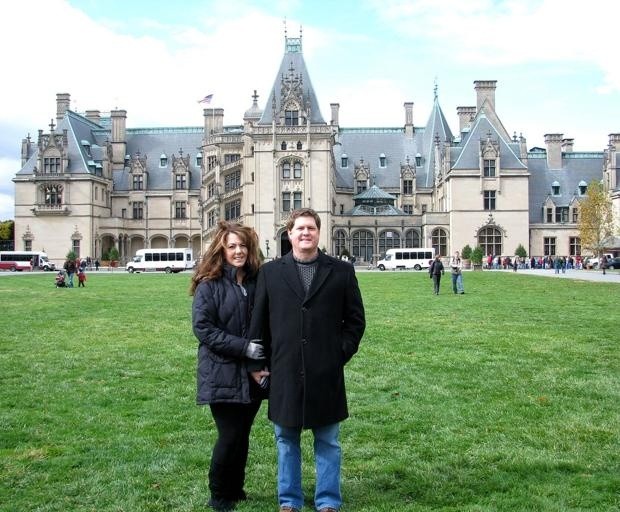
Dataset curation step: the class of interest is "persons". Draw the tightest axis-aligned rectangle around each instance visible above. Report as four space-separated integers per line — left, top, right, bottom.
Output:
245 208 366 511
449 251 465 294
599 255 607 274
189 221 270 511
351 255 356 265
368 257 373 270
55 256 100 288
30 257 34 272
429 254 444 295
111 260 115 272
487 251 587 275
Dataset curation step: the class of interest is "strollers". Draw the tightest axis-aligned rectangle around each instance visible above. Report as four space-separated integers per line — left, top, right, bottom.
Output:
54 270 71 289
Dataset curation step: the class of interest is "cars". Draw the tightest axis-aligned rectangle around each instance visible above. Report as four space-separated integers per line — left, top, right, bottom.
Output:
605 257 620 269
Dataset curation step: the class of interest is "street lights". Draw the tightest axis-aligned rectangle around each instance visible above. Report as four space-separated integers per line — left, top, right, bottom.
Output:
265 239 270 258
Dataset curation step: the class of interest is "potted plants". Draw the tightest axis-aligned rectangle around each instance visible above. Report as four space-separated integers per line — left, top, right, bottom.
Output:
471 245 484 271
100 251 110 266
109 246 120 266
461 244 472 269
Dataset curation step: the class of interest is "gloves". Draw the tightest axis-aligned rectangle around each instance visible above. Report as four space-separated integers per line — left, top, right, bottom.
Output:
245 339 266 359
260 367 271 389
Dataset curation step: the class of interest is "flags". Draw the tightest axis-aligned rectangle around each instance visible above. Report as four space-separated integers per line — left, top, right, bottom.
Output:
198 94 212 105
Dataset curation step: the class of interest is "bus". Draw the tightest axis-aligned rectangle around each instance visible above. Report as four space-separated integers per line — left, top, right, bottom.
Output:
0 250 55 272
124 246 196 273
377 247 436 271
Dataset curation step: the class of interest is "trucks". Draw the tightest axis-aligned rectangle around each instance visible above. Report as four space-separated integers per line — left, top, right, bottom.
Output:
587 254 613 270
